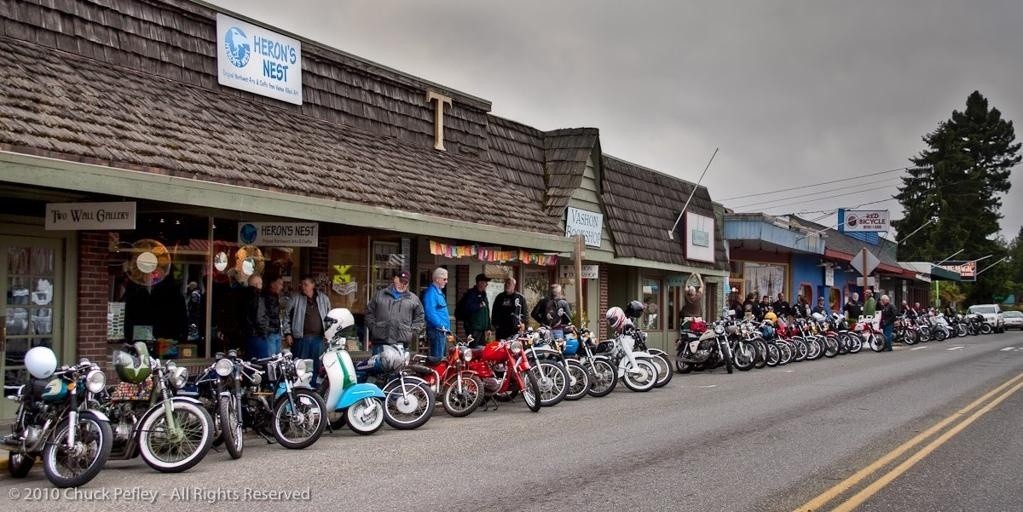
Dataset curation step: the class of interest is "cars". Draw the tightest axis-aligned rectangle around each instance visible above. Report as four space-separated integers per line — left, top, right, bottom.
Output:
1002 311 1023 331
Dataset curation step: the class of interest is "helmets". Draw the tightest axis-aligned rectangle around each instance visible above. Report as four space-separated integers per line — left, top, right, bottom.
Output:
376 342 405 374
25 347 58 377
576 328 599 357
112 342 151 384
626 300 644 318
534 326 552 346
765 313 778 326
324 307 354 341
606 307 627 332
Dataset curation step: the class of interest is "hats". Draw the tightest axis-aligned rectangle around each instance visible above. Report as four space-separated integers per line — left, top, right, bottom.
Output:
395 269 412 283
475 273 490 282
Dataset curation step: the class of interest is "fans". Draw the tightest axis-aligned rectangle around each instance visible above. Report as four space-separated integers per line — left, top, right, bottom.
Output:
116 235 172 288
232 244 263 282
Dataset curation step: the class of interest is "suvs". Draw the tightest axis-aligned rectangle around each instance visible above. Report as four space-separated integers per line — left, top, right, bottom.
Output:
962 304 1004 334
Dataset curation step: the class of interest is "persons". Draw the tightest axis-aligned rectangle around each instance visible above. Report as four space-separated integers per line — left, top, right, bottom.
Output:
197 265 954 363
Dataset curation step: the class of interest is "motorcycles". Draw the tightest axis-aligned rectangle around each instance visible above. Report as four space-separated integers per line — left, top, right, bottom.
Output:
192 299 672 460
40 342 214 473
0 346 113 488
675 300 991 374
